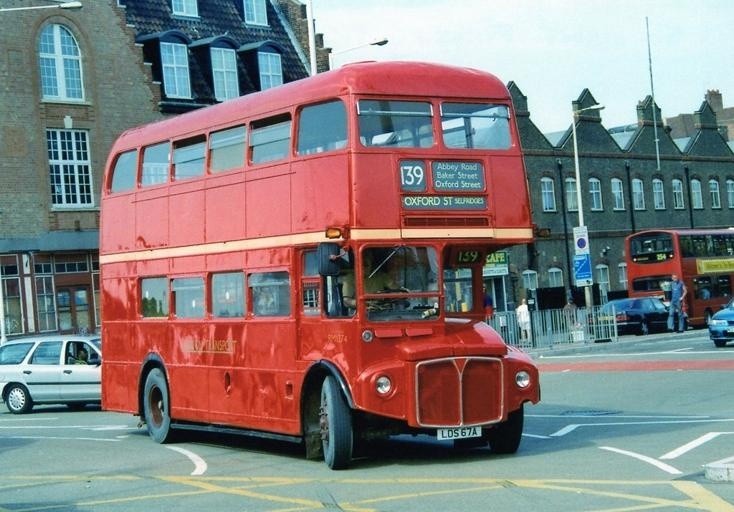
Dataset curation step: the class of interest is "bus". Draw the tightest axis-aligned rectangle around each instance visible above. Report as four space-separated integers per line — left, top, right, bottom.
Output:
99 62 541 470
626 227 734 329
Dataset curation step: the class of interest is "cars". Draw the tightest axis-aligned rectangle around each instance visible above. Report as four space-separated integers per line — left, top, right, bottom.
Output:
588 297 690 337
707 297 734 347
0 335 102 413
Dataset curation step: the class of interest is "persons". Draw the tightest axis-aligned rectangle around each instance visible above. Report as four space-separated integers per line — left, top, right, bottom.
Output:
562 296 579 342
481 283 492 315
516 297 531 344
74 347 89 365
665 272 687 332
700 285 712 300
339 246 408 317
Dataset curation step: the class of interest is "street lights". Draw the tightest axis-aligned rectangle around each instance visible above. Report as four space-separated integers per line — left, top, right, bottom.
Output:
571 104 606 306
306 0 389 75
